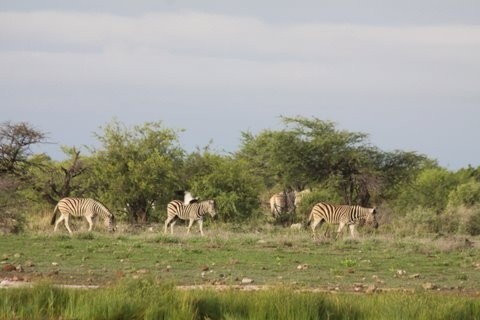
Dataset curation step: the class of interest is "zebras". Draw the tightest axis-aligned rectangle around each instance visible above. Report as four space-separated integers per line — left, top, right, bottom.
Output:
306 202 379 241
164 199 217 236
50 196 115 234
